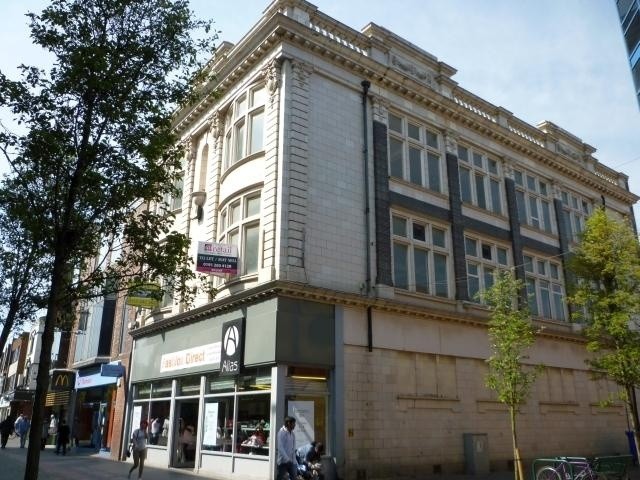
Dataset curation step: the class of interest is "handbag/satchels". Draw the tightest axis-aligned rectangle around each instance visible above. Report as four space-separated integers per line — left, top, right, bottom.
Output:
125 450 130 457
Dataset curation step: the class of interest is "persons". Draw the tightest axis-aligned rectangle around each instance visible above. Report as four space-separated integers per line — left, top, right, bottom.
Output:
275 415 300 480
55 419 71 457
295 440 325 480
0 416 14 448
126 419 148 480
14 411 30 448
150 415 196 462
40 419 49 450
216 419 269 455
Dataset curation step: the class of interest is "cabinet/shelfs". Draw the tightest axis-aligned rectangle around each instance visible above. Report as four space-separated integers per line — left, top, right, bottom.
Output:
240 428 270 450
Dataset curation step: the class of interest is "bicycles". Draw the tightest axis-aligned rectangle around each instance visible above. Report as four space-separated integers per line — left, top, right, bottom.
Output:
537 451 624 479
